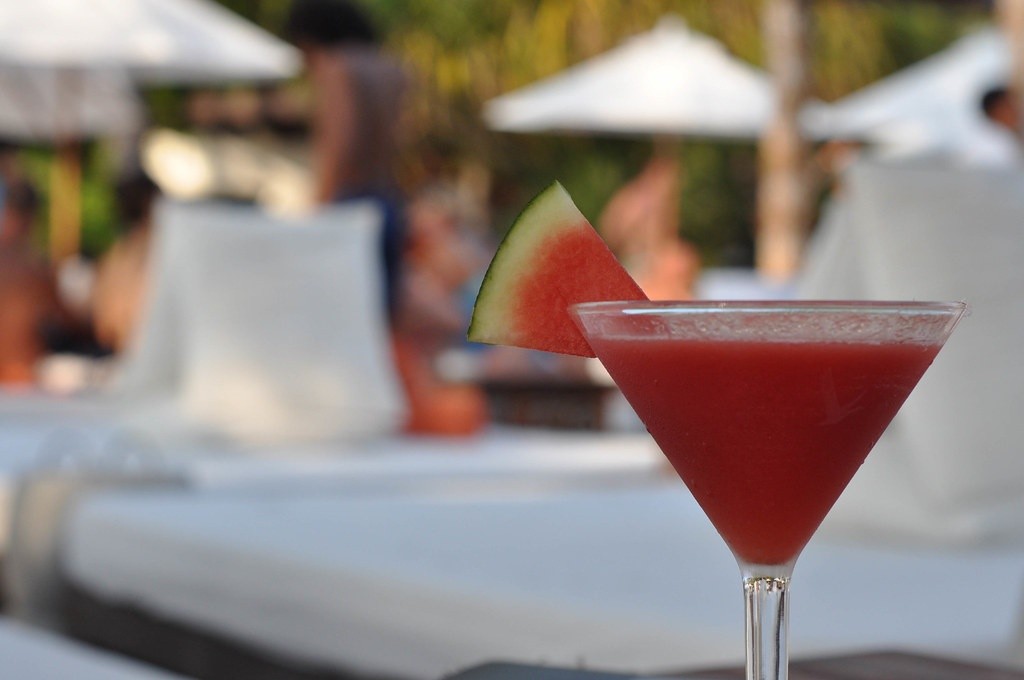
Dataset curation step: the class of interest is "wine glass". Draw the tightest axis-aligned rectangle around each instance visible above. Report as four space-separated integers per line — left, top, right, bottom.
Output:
565 300 969 680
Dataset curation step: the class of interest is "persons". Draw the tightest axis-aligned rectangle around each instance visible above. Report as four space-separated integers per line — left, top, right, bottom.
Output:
281 1 405 318
405 134 702 431
1 136 158 399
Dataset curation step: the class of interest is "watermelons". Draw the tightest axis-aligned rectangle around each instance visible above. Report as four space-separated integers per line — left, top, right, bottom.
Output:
468 183 648 358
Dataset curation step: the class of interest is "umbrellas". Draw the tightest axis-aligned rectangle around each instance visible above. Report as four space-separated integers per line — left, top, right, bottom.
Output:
1 2 304 82
478 10 776 138
